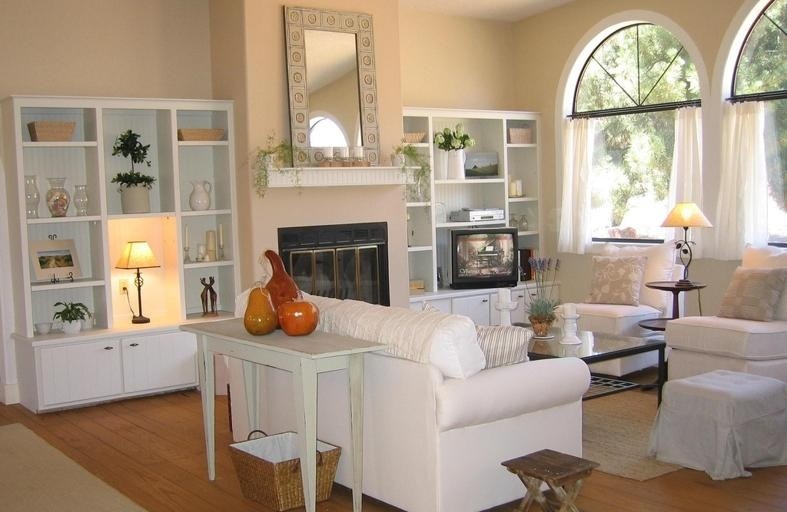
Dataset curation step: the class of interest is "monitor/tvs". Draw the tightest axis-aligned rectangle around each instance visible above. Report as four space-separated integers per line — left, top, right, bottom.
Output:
451 228 518 288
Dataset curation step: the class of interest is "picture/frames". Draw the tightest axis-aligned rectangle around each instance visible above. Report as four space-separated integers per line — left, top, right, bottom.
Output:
26 239 81 280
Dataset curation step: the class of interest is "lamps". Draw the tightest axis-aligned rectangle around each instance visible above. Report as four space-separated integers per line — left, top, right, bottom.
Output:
115 240 161 325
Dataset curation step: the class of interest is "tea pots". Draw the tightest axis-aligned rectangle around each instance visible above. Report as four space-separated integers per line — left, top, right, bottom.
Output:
187 180 213 212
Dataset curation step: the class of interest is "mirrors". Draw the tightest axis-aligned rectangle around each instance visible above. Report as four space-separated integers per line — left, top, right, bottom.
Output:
284 7 380 168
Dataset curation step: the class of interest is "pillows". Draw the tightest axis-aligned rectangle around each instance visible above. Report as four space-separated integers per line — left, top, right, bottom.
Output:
718 266 786 321
421 301 534 369
590 253 645 308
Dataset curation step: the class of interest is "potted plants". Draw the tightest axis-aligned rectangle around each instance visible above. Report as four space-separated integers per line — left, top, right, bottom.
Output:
49 296 92 334
246 131 300 200
114 130 157 215
389 142 430 179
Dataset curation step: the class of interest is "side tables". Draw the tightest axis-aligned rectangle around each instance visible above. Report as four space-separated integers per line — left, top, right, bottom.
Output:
640 278 706 392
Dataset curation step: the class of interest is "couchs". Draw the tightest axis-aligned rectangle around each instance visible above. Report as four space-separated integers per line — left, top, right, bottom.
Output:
228 282 592 509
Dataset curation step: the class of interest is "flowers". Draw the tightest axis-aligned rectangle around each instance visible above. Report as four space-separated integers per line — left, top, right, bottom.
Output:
518 251 562 320
434 119 477 151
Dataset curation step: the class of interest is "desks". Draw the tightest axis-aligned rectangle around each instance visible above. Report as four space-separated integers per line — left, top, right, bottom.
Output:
178 312 386 511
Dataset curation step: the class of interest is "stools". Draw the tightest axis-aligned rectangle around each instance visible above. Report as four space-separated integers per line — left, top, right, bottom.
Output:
655 370 784 477
502 449 599 511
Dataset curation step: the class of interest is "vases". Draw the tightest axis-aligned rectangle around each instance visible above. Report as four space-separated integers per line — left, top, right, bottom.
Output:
530 313 555 336
446 148 466 179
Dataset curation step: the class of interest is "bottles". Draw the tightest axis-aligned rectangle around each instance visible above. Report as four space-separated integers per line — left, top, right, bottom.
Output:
47 176 70 217
26 175 41 219
74 185 90 216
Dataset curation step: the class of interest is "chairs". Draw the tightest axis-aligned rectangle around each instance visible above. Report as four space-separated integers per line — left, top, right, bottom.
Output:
553 242 685 379
667 243 787 383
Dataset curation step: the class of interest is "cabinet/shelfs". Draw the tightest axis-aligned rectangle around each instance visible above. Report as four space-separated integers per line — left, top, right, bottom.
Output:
396 107 564 330
1 93 242 412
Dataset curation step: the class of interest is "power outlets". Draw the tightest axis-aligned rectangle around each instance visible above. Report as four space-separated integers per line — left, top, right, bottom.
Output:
119 278 128 293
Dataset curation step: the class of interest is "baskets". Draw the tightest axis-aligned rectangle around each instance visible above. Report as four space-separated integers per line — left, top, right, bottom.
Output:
28 122 75 141
178 129 223 141
229 430 342 511
507 128 533 144
403 133 426 143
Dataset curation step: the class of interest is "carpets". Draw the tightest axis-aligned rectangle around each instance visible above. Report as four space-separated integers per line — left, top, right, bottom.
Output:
571 384 688 482
0 420 147 512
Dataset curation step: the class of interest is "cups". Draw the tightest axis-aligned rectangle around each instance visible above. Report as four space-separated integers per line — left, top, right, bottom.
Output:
82 315 95 331
35 323 52 335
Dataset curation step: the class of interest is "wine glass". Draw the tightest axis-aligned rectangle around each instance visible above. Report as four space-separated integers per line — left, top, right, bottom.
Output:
508 213 529 231
